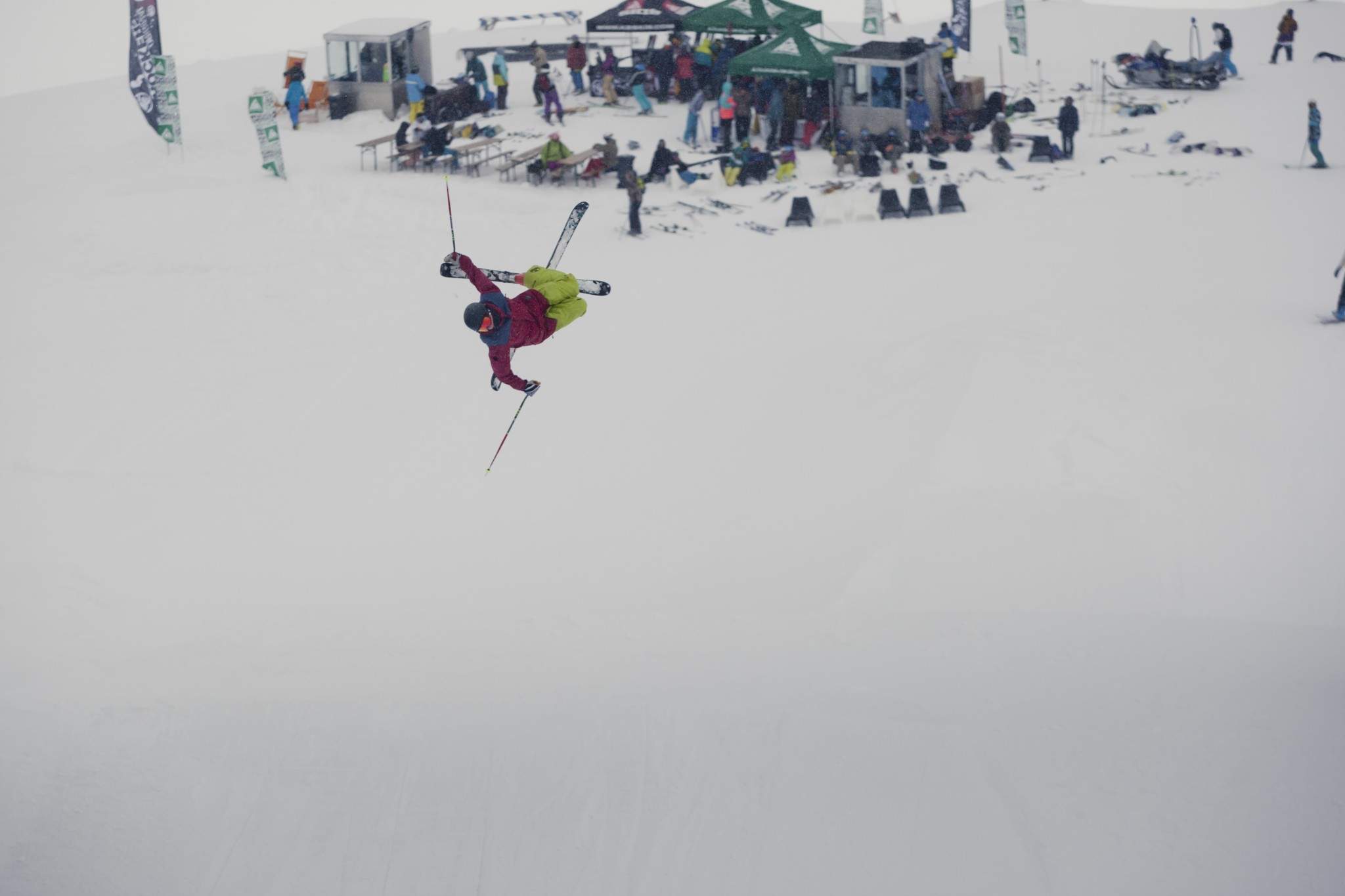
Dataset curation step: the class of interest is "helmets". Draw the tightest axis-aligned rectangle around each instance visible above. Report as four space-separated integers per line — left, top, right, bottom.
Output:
464 303 486 332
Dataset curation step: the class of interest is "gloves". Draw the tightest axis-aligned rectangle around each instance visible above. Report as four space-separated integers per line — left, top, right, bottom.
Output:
524 380 541 397
444 252 462 265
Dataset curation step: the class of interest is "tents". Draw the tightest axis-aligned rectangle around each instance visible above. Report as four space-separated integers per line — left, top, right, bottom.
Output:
585 0 706 74
681 0 824 40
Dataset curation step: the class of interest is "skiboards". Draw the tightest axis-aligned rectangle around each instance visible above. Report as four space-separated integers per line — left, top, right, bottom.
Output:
440 201 610 391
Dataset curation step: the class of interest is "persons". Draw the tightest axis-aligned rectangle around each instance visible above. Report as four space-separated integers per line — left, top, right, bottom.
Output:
1307 99 1327 168
575 131 618 181
1056 95 1080 159
528 39 549 108
282 60 305 89
355 39 462 171
1266 8 1298 64
537 62 564 124
492 47 509 110
442 251 588 396
540 132 573 182
1116 51 1216 74
565 33 588 96
283 73 310 130
1332 251 1345 316
463 50 489 101
622 164 646 235
1211 22 1238 79
614 21 1013 189
595 45 621 108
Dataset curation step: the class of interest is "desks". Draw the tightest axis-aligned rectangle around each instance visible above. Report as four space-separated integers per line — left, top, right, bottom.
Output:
358 129 610 189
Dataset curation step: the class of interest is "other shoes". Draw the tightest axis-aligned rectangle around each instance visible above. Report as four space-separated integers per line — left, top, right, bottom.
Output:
514 274 524 286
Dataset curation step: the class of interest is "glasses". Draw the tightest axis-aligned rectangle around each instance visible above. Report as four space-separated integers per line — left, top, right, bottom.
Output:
477 316 491 332
